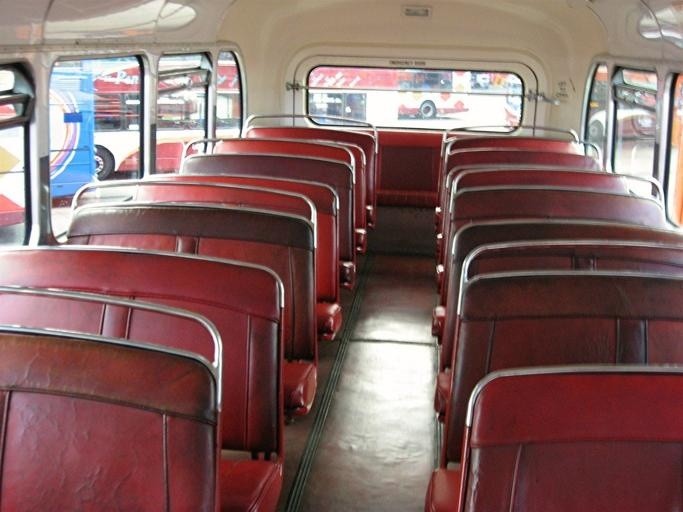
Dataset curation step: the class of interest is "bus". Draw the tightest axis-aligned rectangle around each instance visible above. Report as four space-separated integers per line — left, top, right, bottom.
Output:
504 66 657 143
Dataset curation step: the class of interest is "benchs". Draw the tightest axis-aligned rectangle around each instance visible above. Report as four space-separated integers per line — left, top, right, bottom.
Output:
0 113 378 509
376 124 420 207
423 123 682 512
